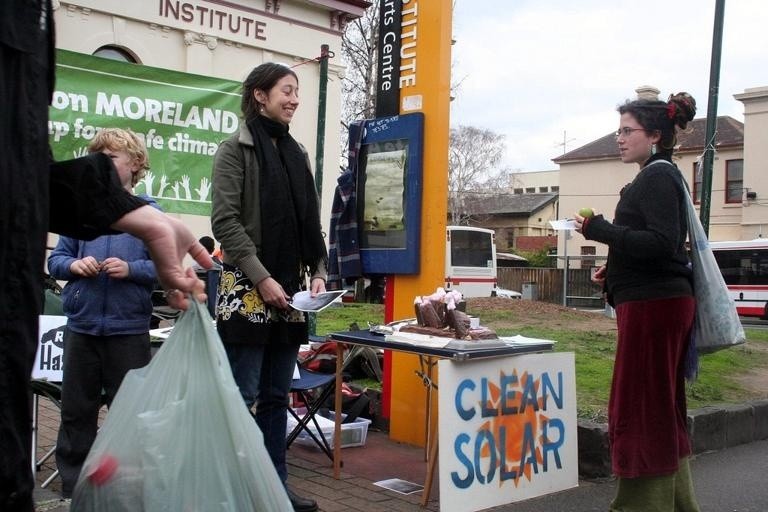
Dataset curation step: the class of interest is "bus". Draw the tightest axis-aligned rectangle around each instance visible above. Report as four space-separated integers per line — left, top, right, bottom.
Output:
687 236 767 322
444 225 498 299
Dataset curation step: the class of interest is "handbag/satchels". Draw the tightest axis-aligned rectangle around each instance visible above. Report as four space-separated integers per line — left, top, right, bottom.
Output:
690 218 748 351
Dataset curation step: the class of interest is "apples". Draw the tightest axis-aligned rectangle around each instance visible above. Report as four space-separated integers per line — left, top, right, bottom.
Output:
577 209 595 224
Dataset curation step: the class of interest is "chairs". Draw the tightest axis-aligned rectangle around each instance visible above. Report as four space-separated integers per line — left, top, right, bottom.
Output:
286 367 352 467
31 290 106 489
152 306 182 327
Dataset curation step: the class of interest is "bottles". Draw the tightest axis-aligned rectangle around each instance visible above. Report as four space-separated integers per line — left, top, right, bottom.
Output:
84 451 148 512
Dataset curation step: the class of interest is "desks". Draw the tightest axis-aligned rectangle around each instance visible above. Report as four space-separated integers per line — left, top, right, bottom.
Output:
330 330 558 507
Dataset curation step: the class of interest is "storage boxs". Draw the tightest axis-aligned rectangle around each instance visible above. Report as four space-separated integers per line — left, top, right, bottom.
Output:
285 406 372 452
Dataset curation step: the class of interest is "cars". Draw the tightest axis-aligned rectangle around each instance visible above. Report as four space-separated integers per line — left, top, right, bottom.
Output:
496 286 521 301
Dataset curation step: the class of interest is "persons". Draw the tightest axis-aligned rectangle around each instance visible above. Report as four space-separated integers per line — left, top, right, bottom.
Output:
572 88 708 511
212 244 225 268
187 234 224 273
45 126 167 511
0 0 220 511
211 61 332 512
